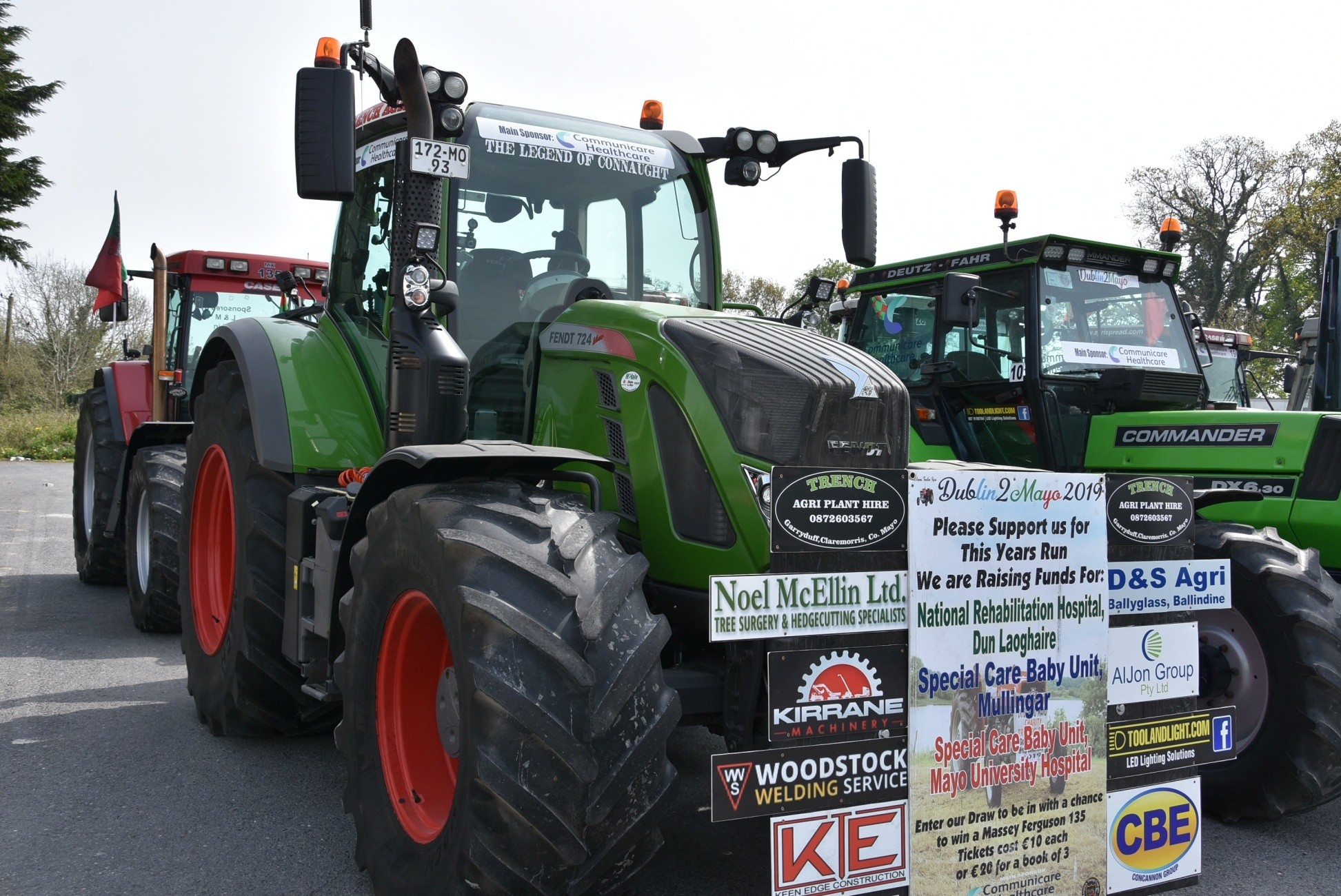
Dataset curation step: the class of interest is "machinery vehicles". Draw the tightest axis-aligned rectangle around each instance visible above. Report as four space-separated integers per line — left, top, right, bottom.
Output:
73 0 1239 896
825 189 1340 824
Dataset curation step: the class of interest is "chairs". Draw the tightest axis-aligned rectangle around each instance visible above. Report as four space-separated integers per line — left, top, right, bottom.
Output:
458 248 533 351
946 351 998 381
